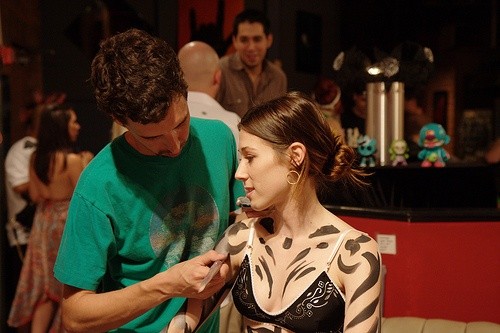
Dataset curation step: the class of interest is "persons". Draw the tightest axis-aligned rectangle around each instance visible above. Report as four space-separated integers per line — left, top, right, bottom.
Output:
217 13 287 119
7 102 94 333
309 79 499 162
178 41 242 163
159 92 382 333
53 30 254 333
4 89 66 272
